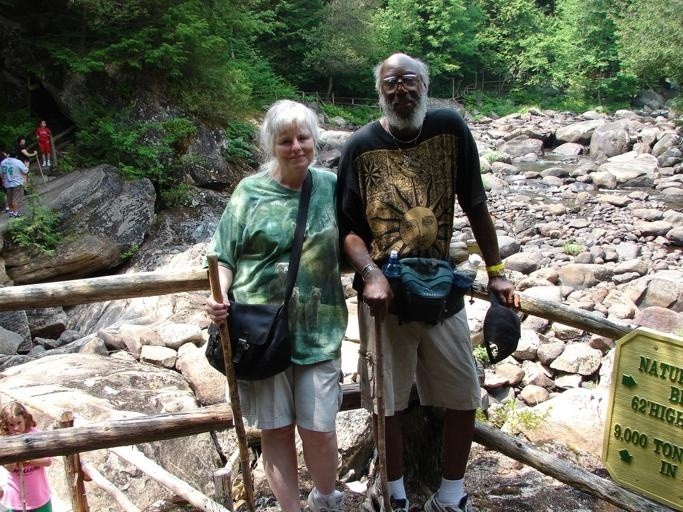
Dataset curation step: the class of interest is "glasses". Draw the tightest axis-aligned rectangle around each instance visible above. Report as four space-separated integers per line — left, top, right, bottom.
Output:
381 74 426 93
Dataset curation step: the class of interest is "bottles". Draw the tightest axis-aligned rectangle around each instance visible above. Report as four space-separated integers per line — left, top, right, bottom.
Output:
381 249 401 282
453 254 484 286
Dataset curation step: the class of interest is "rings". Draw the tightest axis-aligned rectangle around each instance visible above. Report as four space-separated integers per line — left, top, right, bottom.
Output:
508 297 514 301
513 291 519 297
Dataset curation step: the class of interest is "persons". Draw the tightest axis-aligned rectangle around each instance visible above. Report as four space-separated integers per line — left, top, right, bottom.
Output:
35 119 55 167
335 51 521 510
0 401 53 512
0 135 38 217
200 97 350 511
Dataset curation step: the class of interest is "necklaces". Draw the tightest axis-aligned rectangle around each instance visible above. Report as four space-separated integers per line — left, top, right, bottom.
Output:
383 117 426 168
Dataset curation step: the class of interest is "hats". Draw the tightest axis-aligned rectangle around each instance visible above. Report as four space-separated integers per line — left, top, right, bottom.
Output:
484 286 520 364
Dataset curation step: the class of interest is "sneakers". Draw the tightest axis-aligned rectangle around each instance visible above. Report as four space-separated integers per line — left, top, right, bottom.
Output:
380 491 468 512
308 487 347 512
6 207 25 216
42 161 51 166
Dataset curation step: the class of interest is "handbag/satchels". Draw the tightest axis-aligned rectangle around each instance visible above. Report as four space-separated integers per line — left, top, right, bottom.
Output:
353 258 477 324
205 297 290 380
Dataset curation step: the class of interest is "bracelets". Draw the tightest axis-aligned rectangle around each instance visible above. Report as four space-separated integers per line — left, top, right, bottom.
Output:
360 264 378 282
487 264 506 273
